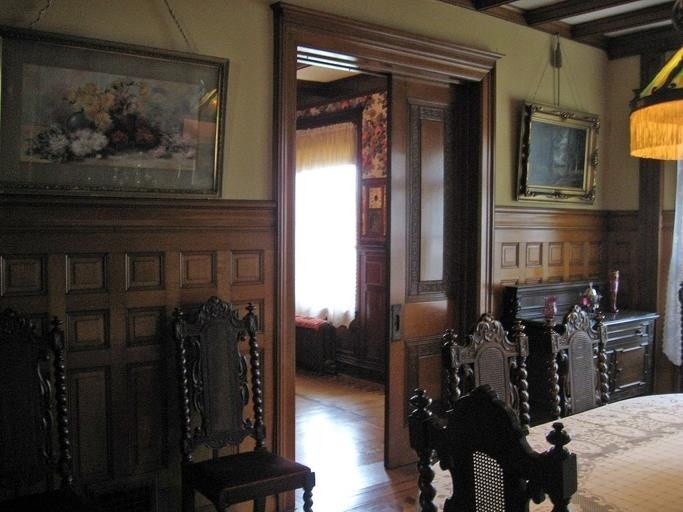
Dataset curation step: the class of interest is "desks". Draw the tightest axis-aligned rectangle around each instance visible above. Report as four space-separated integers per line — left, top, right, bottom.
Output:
417 392 683 512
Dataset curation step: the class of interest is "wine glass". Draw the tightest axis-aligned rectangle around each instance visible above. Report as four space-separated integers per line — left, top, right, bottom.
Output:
607 268 620 313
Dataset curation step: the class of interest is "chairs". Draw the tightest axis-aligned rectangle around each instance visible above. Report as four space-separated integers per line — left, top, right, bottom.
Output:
0 306 96 512
172 294 315 512
442 313 530 429
407 383 578 512
543 304 610 421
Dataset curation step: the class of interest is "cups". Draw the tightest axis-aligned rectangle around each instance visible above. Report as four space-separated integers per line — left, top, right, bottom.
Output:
542 295 557 319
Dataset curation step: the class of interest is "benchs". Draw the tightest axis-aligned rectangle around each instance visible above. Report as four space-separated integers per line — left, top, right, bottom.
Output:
296 314 339 377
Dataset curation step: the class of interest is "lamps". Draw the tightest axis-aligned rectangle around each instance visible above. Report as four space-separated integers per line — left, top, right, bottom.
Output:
628 45 683 161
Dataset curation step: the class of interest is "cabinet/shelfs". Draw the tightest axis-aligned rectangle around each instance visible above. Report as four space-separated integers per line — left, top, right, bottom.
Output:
502 278 660 428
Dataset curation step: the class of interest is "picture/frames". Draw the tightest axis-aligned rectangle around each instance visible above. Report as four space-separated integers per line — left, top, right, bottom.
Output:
515 98 600 205
0 26 230 200
